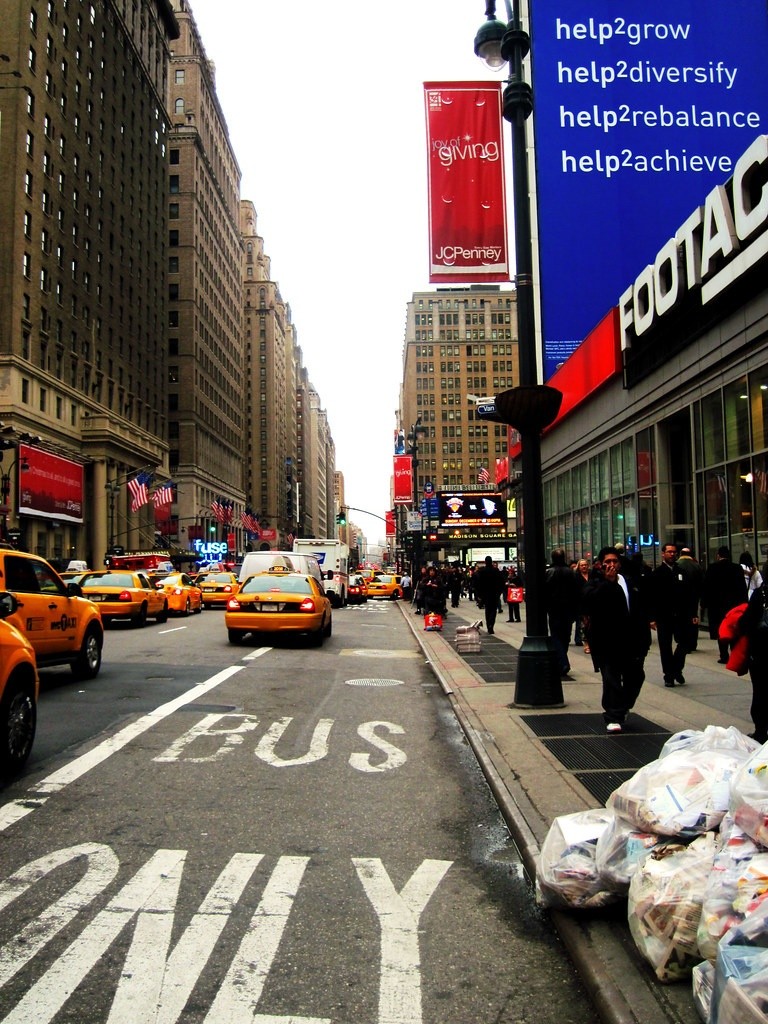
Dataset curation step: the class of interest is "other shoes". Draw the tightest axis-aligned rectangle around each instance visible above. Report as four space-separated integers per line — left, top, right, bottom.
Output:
675 671 686 684
515 619 521 622
665 675 674 687
584 645 590 653
506 619 514 622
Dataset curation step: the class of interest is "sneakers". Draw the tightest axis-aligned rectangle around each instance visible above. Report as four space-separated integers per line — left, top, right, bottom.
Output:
606 723 622 730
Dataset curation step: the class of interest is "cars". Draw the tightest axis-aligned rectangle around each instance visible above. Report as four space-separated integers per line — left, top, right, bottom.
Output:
193 565 241 608
41 560 96 591
79 573 168 626
1 549 105 774
226 553 333 646
347 569 408 602
154 570 203 616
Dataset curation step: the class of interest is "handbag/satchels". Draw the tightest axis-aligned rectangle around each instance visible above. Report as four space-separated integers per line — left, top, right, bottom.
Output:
507 584 523 603
424 611 444 631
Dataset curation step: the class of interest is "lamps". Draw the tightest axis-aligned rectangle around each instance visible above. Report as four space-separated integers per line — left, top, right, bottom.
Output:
0 425 42 444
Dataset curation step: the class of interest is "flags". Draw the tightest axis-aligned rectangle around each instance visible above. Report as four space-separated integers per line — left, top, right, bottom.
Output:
127 469 173 514
240 508 262 536
287 533 294 543
211 496 234 525
755 468 768 495
477 467 490 484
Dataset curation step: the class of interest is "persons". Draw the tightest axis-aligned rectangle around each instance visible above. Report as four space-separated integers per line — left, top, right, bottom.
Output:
471 556 506 634
545 542 763 688
400 572 411 600
584 546 652 731
730 549 768 745
414 560 524 622
394 430 404 454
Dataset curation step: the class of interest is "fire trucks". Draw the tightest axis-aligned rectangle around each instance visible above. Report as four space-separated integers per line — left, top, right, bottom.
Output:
112 556 169 571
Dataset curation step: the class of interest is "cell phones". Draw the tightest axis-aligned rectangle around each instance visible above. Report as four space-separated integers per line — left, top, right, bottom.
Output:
601 564 619 574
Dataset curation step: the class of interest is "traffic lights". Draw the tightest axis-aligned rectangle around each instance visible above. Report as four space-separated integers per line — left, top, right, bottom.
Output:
211 521 216 532
335 512 347 526
362 557 365 562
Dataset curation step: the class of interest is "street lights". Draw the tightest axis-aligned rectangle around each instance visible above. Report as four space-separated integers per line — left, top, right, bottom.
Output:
0 453 30 548
181 526 193 571
398 415 427 583
105 479 120 555
476 0 565 708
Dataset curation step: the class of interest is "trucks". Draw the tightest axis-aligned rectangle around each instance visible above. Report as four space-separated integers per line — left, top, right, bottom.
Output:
294 539 355 604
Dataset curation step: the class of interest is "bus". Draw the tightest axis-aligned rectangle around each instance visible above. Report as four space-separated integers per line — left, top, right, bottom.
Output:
348 548 359 571
